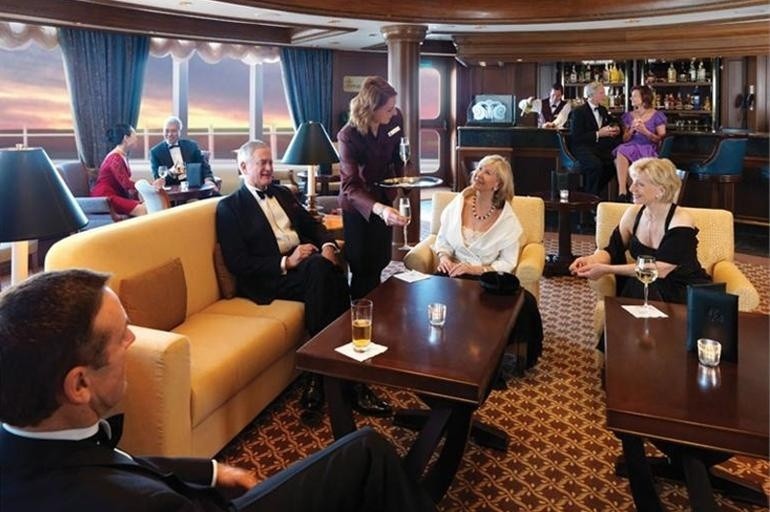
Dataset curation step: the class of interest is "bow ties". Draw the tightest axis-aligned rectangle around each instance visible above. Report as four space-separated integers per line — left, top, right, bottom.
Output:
256 188 274 199
169 145 180 148
102 413 125 450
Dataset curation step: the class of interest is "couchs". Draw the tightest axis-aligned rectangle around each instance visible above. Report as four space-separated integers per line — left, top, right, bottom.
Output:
44 195 306 459
211 159 341 193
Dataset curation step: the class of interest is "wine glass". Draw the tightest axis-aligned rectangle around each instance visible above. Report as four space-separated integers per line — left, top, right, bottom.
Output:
158 166 168 191
633 255 659 314
398 197 414 250
675 119 711 132
636 308 657 352
398 136 411 182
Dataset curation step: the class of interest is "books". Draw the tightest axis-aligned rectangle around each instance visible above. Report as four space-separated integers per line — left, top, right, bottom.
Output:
186 163 205 189
686 282 739 364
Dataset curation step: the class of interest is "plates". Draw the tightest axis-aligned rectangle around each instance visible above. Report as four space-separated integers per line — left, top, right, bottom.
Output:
379 175 443 188
722 128 746 133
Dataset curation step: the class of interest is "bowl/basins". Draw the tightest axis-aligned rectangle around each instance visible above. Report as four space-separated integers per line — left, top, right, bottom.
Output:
479 271 520 296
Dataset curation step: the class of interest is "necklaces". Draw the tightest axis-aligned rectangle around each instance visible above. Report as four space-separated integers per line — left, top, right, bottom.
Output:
117 147 130 158
471 195 496 221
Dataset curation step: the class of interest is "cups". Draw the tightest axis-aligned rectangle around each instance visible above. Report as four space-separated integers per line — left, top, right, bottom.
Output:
350 298 373 353
697 337 722 367
180 181 189 190
429 326 447 344
696 363 721 389
560 189 569 199
427 302 447 326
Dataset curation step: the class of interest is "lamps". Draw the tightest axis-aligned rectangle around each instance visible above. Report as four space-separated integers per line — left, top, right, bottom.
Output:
0 144 89 285
281 120 340 223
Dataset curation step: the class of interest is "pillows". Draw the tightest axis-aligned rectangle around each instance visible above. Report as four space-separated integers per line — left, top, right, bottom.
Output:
212 239 235 301
118 255 188 330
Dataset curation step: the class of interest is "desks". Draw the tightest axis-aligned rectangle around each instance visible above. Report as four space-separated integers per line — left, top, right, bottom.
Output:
295 170 340 194
530 189 602 274
321 214 344 241
164 180 216 201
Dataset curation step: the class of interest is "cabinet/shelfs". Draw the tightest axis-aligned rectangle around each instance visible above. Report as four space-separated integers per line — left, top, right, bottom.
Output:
556 58 630 120
640 57 720 131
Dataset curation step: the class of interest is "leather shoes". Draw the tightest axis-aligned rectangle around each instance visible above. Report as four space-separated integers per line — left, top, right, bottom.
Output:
301 377 324 413
617 189 629 202
349 382 392 417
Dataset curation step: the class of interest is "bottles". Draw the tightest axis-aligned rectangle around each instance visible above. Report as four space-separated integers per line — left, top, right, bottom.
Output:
645 61 712 111
565 64 624 110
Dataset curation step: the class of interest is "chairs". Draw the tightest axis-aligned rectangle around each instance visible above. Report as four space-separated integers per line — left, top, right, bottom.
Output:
587 201 760 370
201 151 222 191
37 159 115 266
402 190 547 376
690 137 750 175
555 130 582 170
134 178 170 214
656 136 674 158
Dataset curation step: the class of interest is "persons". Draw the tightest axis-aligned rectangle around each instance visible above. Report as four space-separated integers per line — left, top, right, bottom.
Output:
568 157 715 391
149 117 220 205
0 268 428 511
611 85 668 202
90 123 148 218
216 139 391 417
336 75 411 304
563 81 622 196
518 83 571 129
429 154 524 282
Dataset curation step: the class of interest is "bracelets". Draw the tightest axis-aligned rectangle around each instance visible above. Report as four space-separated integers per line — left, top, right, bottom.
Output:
438 254 449 259
483 265 487 273
379 206 387 219
